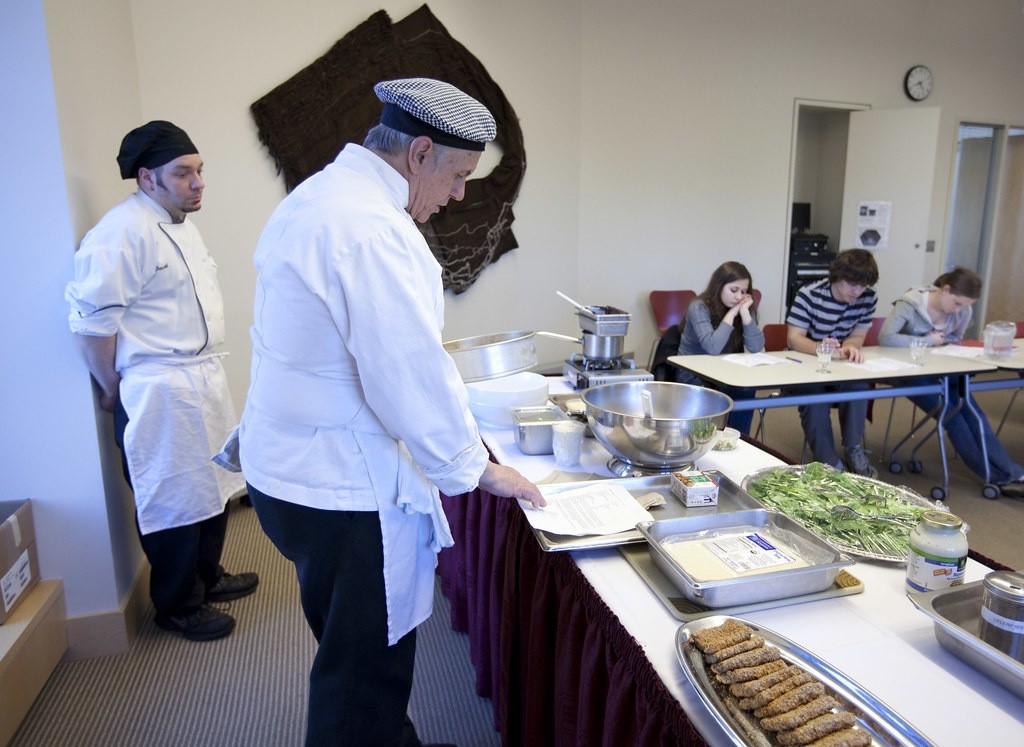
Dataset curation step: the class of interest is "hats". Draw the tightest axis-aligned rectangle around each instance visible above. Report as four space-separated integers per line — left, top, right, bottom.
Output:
373 76 496 153
116 118 198 179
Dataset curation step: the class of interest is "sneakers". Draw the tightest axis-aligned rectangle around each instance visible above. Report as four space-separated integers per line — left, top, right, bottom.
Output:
844 444 878 480
205 566 259 603
154 595 235 641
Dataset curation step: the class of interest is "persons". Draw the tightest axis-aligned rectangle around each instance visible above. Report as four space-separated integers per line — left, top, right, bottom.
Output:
64 120 259 641
879 267 1024 498
213 76 548 747
783 247 879 480
673 261 766 436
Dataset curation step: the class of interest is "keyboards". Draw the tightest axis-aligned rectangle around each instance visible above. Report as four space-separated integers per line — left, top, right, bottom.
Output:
798 252 835 263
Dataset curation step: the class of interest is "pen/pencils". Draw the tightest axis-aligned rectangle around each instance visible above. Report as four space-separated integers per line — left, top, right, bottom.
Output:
786 357 802 363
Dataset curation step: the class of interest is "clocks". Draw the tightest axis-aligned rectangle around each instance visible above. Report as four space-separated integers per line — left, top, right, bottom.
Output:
906 66 933 100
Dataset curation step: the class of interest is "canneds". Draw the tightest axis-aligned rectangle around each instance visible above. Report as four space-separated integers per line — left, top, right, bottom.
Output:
906 510 969 595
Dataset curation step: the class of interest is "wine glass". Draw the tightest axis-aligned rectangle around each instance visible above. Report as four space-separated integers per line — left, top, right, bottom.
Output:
908 336 929 367
815 340 834 374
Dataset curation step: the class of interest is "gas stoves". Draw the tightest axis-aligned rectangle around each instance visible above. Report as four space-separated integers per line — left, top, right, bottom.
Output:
562 352 655 388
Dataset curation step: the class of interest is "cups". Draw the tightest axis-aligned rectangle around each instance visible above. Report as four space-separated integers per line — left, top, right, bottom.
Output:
978 570 1024 665
982 325 1012 360
552 420 585 467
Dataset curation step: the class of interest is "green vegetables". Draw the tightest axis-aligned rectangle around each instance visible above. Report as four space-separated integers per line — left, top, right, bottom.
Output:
746 462 924 550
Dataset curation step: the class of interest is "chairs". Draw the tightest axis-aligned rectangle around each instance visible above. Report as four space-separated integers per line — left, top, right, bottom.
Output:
755 324 808 465
647 290 697 371
862 318 916 462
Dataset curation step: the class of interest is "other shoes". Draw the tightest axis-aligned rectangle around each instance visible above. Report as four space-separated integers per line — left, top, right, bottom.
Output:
999 470 1024 495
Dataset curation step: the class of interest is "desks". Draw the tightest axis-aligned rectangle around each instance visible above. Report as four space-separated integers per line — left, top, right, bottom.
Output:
439 376 1024 747
666 338 1024 500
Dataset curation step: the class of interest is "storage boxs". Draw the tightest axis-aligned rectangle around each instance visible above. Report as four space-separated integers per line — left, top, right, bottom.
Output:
0 498 41 626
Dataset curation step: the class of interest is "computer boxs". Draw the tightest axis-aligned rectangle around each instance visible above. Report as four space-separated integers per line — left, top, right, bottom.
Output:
791 235 827 252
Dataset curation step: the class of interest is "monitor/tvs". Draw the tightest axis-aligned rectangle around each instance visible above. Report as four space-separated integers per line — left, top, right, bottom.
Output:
792 202 811 234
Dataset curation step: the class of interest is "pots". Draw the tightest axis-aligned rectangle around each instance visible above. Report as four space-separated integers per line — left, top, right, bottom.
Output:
535 330 624 360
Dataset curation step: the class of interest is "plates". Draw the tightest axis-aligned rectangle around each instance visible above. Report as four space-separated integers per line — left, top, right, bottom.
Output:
741 464 950 562
675 614 939 747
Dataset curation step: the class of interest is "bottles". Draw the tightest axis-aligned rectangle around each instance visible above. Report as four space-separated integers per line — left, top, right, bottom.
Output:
905 510 968 594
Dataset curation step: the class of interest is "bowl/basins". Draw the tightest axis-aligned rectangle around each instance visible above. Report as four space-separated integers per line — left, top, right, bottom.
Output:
580 381 735 478
712 427 740 450
464 371 549 428
